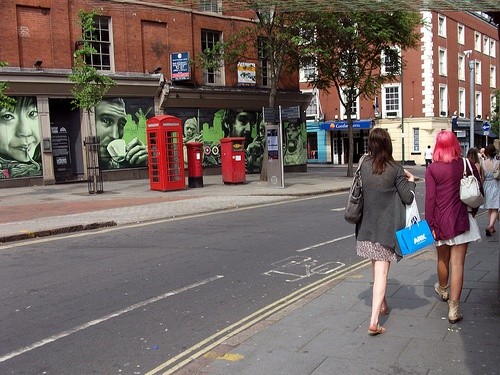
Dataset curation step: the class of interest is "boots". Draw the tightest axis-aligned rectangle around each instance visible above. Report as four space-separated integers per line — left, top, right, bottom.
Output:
446 299 464 324
434 283 450 302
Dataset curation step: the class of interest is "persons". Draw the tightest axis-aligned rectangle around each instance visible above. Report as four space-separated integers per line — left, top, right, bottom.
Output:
424 145 433 170
0 96 41 163
425 130 486 324
95 97 148 167
344 127 434 335
467 145 486 218
165 108 306 164
482 144 500 236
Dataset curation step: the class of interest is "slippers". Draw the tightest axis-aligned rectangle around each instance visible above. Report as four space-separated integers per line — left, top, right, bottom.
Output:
368 326 386 334
380 305 389 315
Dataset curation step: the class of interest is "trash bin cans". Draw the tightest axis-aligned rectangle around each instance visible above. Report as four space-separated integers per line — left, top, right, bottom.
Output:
220 137 246 183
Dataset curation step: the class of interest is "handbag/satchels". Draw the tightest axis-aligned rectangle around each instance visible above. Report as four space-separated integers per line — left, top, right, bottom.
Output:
460 157 485 209
395 219 435 256
492 163 500 181
405 190 421 228
345 176 363 224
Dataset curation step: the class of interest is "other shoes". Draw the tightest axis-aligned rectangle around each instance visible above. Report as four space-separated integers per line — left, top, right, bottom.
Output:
485 229 492 237
